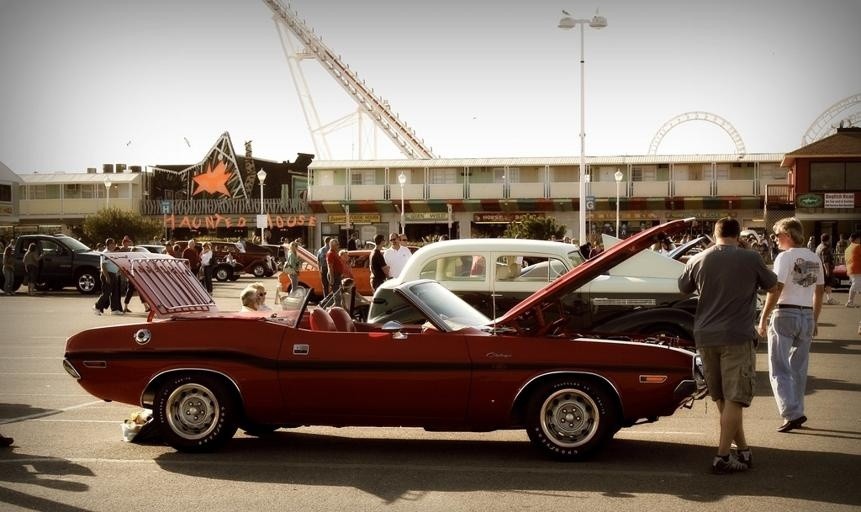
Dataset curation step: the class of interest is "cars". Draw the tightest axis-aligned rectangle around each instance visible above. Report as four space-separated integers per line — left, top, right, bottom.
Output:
279 243 373 296
367 238 700 336
168 240 278 282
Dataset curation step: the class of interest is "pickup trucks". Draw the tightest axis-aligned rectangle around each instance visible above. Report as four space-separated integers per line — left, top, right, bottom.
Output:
0 234 102 294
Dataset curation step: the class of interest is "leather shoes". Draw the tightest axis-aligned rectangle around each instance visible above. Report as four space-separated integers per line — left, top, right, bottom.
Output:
124 309 131 314
144 307 152 313
777 415 806 433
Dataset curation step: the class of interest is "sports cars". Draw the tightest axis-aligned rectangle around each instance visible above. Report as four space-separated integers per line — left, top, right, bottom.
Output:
63 217 709 462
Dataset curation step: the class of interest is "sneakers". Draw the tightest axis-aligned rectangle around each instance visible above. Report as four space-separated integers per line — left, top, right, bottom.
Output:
844 303 860 308
4 290 16 296
736 447 754 468
111 309 123 315
27 292 36 296
711 453 748 473
92 304 101 315
827 299 841 305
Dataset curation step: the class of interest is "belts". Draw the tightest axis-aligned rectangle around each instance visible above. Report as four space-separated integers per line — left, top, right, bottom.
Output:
775 302 814 312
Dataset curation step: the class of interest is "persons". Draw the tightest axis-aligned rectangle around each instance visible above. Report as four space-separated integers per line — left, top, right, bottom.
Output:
326 238 346 306
22 243 46 297
317 236 332 300
845 231 861 308
240 290 260 312
806 235 816 251
547 234 604 260
94 242 106 252
165 243 176 257
2 238 18 296
92 238 124 315
816 233 840 307
199 243 215 297
119 237 134 313
677 217 779 475
834 233 848 258
172 244 182 259
282 238 300 296
769 233 776 260
347 233 358 250
279 236 290 260
384 232 413 279
766 217 825 433
398 233 409 247
153 235 161 245
181 240 196 264
835 233 847 263
251 283 272 311
298 237 306 246
370 234 390 292
342 277 370 321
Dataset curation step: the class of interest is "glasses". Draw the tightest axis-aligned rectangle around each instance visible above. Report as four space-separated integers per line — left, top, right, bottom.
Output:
389 237 399 242
400 238 408 242
109 243 116 245
258 291 267 297
774 233 784 237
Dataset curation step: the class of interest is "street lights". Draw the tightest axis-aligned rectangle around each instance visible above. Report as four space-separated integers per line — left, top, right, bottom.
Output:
255 167 269 246
257 167 269 246
101 175 115 209
103 175 113 212
398 171 408 235
397 169 410 236
